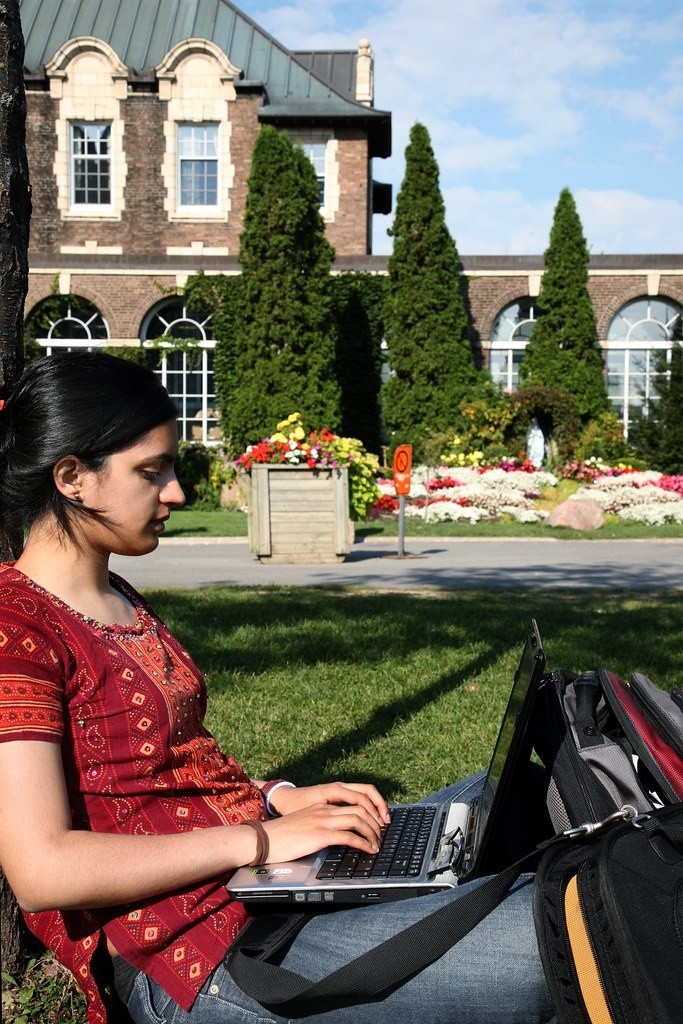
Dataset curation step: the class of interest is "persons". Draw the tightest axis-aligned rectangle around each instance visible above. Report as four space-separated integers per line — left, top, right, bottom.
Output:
0 353 555 1024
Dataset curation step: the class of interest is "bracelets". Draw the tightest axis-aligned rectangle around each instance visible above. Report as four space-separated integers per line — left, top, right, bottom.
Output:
260 779 296 816
241 820 269 866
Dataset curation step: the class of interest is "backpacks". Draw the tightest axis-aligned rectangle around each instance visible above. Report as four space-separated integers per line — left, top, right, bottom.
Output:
532 802 683 1024
533 669 683 840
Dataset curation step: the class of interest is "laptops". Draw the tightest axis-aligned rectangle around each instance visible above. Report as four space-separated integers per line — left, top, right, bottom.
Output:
224 619 545 903
